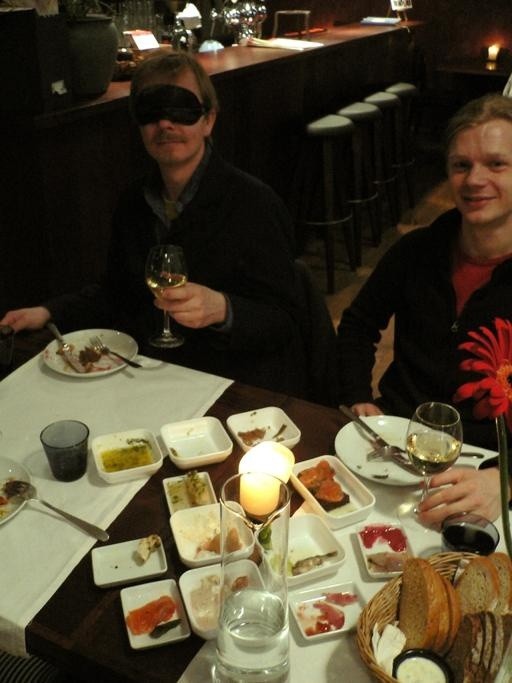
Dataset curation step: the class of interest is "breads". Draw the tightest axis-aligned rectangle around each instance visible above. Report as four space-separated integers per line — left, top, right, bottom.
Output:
398 552 512 683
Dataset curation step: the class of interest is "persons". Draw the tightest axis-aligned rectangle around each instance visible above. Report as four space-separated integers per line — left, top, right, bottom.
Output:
1 51 298 386
328 94 512 532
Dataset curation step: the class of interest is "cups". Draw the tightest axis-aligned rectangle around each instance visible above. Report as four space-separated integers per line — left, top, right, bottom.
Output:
441 512 500 555
38 417 88 481
214 471 290 682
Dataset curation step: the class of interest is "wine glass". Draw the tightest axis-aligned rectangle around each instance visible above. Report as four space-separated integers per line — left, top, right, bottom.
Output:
144 243 187 347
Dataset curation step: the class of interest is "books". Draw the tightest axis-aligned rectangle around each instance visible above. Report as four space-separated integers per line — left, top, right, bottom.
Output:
360 16 401 26
246 38 323 50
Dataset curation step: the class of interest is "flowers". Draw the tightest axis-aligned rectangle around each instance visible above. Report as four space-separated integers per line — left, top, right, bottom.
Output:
452 319 512 559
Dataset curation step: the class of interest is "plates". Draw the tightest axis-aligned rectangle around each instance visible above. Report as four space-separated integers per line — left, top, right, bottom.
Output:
179 558 267 642
257 513 348 587
170 500 256 566
223 404 303 453
289 580 370 643
0 453 30 526
120 579 190 649
91 535 168 589
290 453 376 528
90 428 163 483
162 471 217 515
356 520 414 580
335 414 440 487
40 327 138 377
161 416 233 471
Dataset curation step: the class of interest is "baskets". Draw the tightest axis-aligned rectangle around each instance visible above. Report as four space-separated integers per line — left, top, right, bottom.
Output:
354 549 485 682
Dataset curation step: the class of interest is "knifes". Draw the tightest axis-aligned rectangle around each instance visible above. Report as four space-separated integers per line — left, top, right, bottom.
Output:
46 319 85 376
324 395 421 474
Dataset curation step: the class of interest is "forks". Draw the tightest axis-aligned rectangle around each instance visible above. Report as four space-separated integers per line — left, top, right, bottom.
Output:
3 476 112 543
360 444 486 461
90 336 142 368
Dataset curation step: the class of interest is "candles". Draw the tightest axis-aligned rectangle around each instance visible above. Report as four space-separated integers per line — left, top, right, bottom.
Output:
238 466 284 515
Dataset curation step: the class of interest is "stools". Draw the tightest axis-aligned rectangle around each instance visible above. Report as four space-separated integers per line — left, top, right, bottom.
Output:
280 79 417 290
